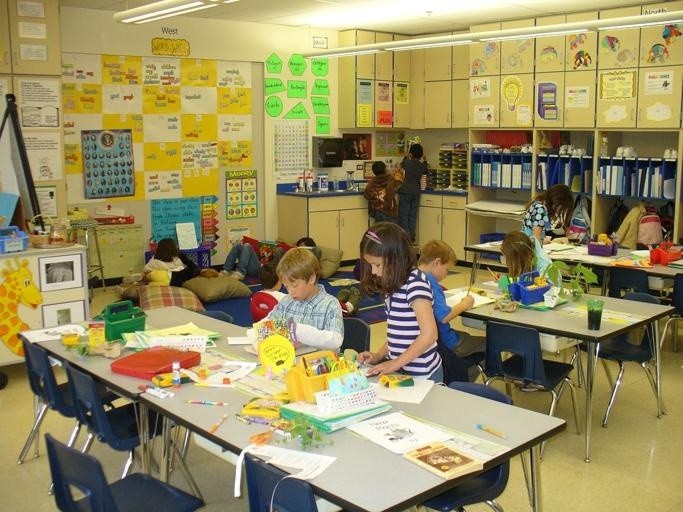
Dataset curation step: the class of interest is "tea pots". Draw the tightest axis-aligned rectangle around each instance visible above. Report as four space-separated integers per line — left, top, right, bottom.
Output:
614 144 638 158
559 143 586 156
502 144 531 155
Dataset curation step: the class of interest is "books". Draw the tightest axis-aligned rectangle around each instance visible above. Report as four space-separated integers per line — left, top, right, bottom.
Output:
474 161 675 199
403 442 484 481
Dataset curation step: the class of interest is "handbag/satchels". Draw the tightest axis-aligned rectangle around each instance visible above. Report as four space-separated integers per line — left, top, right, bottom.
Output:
112 346 200 379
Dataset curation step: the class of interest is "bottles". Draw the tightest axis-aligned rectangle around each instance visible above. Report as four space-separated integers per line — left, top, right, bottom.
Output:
294 186 298 192
298 175 304 192
305 176 313 193
171 360 180 388
50 224 69 245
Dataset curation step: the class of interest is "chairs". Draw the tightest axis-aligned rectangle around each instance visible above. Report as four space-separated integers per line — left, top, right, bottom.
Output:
244 452 319 511
16 333 123 496
63 360 193 480
43 431 206 511
340 316 370 354
558 292 671 428
420 381 513 512
483 320 581 462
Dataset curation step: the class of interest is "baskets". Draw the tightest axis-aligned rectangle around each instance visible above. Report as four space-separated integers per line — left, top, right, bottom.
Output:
29 214 54 245
314 383 379 414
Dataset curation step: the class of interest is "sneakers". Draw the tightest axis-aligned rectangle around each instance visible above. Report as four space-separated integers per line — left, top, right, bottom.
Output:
616 146 638 158
664 149 677 158
559 144 586 156
510 144 533 153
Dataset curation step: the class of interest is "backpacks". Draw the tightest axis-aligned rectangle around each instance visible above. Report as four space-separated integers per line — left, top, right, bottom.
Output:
546 195 590 243
607 202 674 250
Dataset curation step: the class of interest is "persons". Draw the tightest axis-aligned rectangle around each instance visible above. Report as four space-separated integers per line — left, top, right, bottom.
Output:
501 232 562 394
397 144 428 242
247 245 345 355
143 237 361 316
364 160 403 224
521 184 573 249
418 239 486 381
360 221 443 384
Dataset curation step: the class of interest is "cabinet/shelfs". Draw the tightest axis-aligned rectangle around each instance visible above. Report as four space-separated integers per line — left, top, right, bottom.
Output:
82 226 143 282
467 131 682 278
338 1 682 131
309 196 370 263
417 195 467 267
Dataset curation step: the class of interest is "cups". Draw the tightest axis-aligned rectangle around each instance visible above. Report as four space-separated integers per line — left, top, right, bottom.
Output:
662 149 677 159
585 298 604 331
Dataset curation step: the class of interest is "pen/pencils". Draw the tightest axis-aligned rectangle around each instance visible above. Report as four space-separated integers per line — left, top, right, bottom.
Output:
466 287 471 297
486 266 500 282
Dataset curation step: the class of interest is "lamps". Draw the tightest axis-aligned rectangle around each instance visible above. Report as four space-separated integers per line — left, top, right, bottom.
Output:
301 10 683 60
112 0 236 26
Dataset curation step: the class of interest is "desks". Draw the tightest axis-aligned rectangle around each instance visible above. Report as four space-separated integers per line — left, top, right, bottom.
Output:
17 305 321 475
139 350 567 511
443 279 676 463
463 241 682 353
0 240 91 368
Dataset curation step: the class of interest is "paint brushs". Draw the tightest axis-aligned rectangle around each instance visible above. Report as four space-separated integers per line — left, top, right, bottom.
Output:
648 244 653 251
209 413 229 434
139 384 166 399
257 318 297 340
186 400 230 407
235 416 250 424
149 385 175 398
306 357 345 374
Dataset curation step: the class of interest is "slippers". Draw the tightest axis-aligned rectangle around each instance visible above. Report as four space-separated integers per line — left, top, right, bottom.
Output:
337 287 361 316
